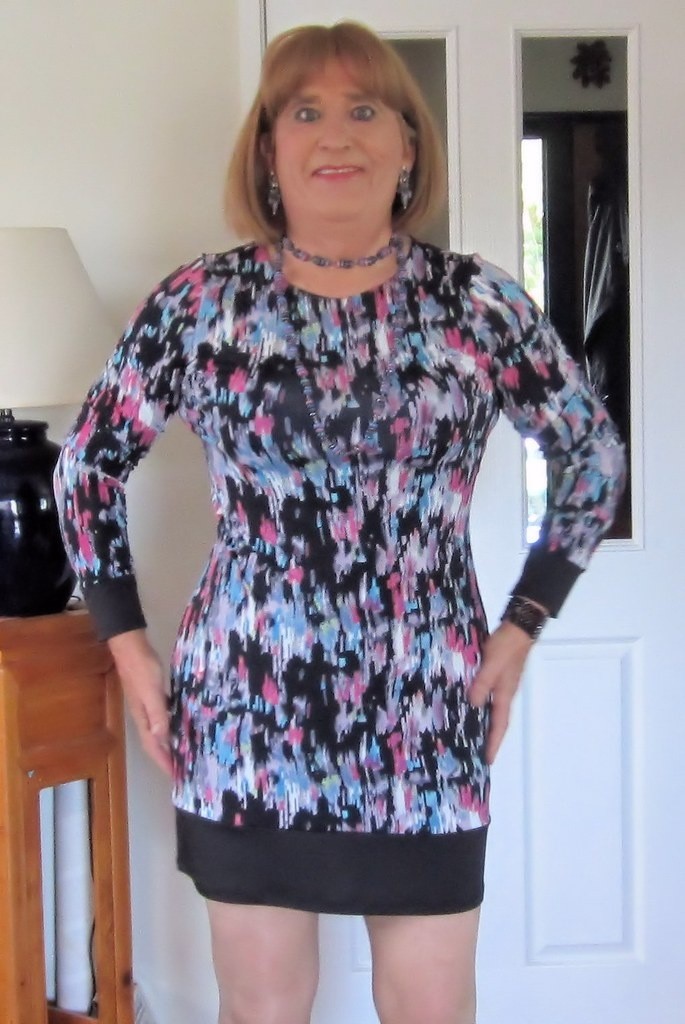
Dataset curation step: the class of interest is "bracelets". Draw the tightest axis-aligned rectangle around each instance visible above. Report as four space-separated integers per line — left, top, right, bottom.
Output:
500 596 550 642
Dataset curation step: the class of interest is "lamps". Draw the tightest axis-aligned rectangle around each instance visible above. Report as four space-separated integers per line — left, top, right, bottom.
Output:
0 225 121 617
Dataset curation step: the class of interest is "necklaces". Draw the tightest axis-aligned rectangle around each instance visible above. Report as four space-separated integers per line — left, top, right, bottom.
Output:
271 235 406 458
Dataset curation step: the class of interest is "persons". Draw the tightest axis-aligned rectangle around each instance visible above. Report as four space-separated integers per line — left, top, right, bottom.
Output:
51 21 628 1024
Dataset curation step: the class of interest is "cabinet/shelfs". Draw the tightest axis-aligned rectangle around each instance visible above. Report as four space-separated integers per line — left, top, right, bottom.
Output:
0 603 134 1024
